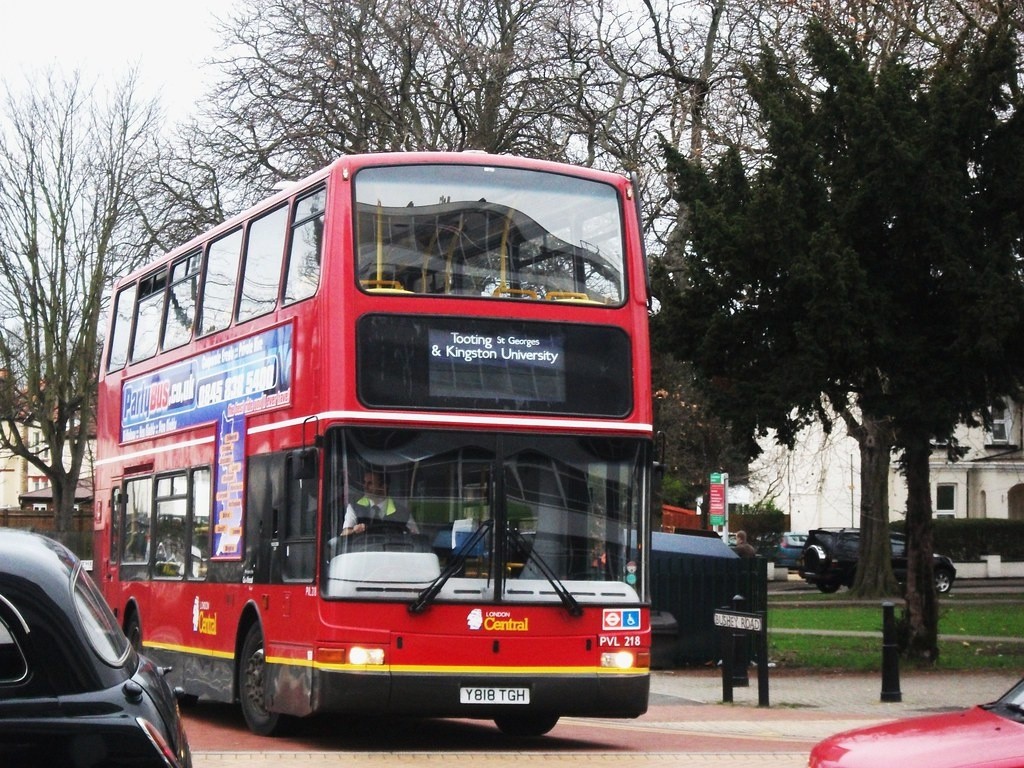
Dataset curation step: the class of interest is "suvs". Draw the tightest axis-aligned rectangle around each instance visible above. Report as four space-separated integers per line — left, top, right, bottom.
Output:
797 527 957 596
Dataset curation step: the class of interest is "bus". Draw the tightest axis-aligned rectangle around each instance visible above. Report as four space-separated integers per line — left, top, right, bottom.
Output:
91 148 655 742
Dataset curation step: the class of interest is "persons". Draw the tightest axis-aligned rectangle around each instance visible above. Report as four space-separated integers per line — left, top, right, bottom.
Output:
731 530 755 557
341 469 419 536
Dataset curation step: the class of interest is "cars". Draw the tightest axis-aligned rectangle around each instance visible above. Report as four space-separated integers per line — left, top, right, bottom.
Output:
0 526 195 768
807 677 1024 768
757 531 807 570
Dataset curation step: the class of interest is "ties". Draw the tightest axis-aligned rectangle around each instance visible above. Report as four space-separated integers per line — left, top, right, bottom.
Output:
371 505 382 525
